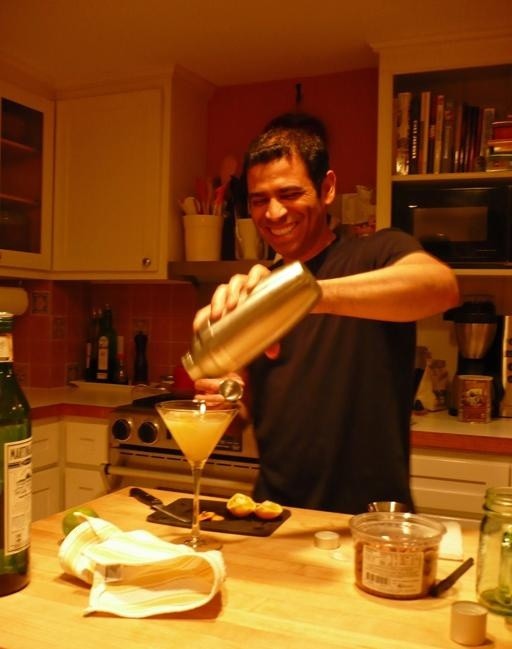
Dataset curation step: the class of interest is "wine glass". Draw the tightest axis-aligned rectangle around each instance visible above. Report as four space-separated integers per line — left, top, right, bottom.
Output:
155 400 240 554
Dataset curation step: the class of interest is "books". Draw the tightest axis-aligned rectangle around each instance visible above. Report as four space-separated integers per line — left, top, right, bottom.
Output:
390 90 512 174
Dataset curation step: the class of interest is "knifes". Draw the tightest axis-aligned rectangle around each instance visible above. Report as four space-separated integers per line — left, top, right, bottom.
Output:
128 488 192 524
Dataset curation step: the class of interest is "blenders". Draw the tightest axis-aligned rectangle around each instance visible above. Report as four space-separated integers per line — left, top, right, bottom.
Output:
443 302 499 414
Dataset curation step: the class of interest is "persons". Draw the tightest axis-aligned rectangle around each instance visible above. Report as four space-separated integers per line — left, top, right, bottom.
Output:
190 123 466 516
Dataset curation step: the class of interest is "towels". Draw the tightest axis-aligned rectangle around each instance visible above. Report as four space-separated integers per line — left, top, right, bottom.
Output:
58 512 226 618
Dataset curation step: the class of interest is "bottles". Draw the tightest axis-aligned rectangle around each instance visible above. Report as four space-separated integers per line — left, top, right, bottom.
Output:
0 310 35 597
178 260 323 381
477 488 512 614
84 303 149 383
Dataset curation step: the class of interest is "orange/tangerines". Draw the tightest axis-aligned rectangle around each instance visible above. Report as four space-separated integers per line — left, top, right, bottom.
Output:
63 508 98 536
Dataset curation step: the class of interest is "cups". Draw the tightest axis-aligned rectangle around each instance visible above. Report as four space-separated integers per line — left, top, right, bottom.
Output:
347 509 446 599
235 218 264 259
160 365 176 385
457 374 492 424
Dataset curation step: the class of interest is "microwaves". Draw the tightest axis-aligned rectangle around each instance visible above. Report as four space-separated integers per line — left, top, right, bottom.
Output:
395 186 509 264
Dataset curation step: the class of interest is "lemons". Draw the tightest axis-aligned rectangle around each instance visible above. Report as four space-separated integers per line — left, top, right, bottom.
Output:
256 498 283 518
226 492 256 517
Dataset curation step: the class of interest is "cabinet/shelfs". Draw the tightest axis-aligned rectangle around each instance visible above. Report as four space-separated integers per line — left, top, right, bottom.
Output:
54 76 214 285
0 78 57 280
375 37 512 276
30 422 62 524
409 454 511 520
62 422 110 510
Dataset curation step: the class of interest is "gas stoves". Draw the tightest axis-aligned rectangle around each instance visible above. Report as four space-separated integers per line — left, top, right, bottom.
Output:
108 391 258 460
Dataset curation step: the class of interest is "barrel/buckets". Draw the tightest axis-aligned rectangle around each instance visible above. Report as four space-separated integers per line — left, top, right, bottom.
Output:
184 214 225 261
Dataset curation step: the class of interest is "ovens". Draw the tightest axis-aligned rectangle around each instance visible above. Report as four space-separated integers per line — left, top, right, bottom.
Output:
101 448 261 499
